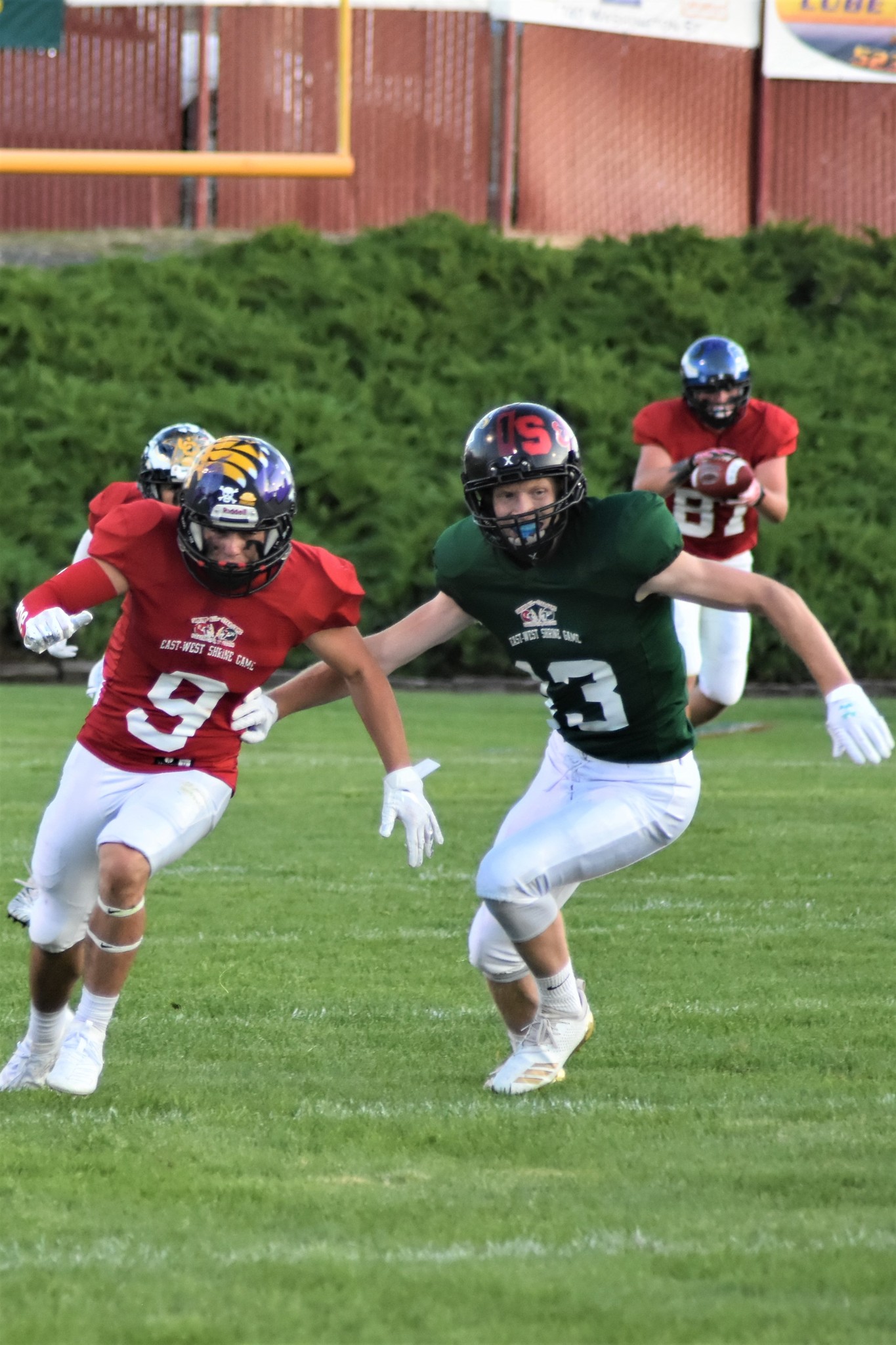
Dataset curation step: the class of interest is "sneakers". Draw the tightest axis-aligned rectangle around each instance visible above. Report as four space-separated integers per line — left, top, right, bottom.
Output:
47 1014 104 1095
0 1006 75 1094
482 977 595 1093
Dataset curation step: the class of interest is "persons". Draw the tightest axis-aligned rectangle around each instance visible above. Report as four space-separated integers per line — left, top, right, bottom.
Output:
229 402 896 1096
0 435 444 1095
631 335 799 728
8 422 214 927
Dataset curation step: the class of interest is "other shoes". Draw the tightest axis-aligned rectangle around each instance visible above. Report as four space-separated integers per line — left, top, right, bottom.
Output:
6 875 34 926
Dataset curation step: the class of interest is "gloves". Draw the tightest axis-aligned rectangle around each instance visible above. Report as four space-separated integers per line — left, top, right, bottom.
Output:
824 684 895 766
233 687 279 744
379 757 448 870
25 606 93 655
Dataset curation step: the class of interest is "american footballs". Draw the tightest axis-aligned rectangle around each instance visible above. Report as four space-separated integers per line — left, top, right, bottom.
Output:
690 455 755 498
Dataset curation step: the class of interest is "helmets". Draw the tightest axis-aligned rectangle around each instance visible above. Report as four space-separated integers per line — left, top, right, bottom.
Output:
460 402 587 568
137 422 216 504
681 336 751 433
178 435 295 599
